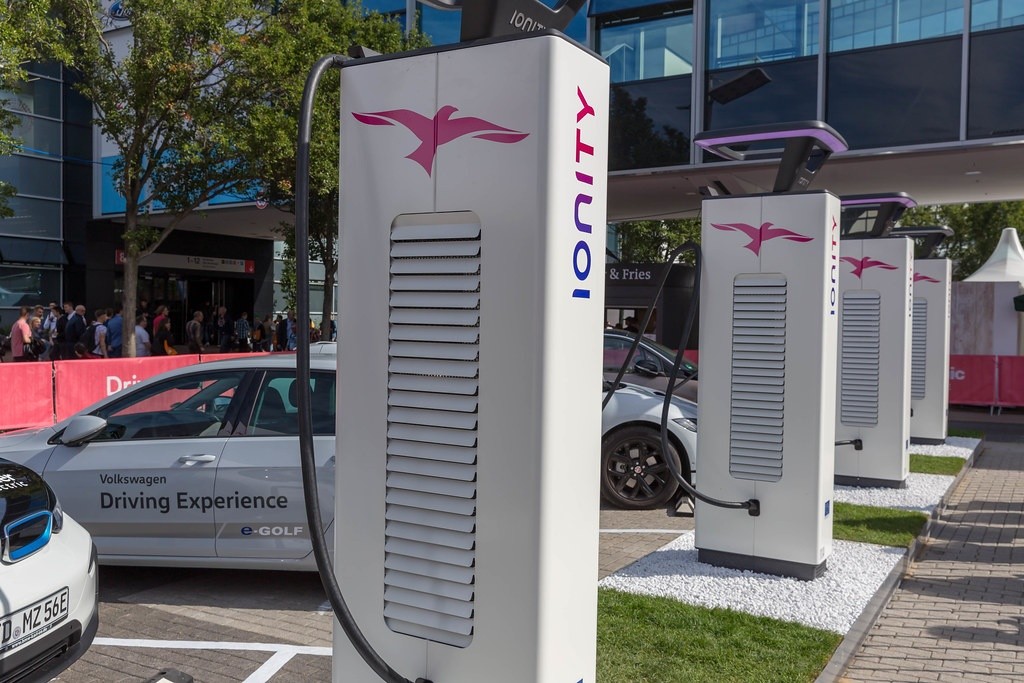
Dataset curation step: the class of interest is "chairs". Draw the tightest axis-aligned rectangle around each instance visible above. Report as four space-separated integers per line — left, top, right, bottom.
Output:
256 387 298 434
288 379 332 415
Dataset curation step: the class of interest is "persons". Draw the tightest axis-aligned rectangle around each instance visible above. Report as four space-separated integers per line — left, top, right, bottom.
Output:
616 317 640 334
251 315 273 352
272 311 296 351
31 302 122 360
135 300 174 356
330 315 337 341
30 318 41 339
202 299 217 346
310 318 320 343
186 311 205 354
215 306 235 352
236 312 251 352
10 306 32 361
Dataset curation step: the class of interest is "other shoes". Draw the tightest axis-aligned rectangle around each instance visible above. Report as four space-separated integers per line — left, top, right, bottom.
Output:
204 342 209 349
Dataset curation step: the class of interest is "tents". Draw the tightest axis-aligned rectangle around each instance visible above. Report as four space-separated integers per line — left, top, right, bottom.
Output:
962 228 1024 288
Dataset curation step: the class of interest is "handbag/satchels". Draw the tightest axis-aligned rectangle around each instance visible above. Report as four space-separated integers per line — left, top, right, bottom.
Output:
164 339 177 355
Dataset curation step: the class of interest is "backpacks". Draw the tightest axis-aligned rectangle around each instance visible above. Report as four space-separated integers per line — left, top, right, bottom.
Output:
80 324 101 354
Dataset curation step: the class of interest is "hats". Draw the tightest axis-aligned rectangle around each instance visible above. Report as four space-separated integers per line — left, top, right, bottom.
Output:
95 310 106 317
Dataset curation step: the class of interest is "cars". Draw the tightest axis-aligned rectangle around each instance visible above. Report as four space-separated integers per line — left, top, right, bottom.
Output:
600 378 700 509
601 328 699 405
0 457 100 683
0 350 338 577
